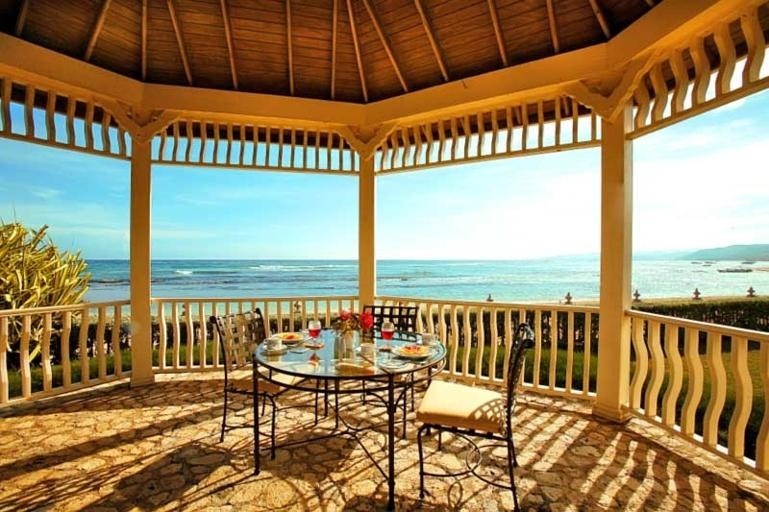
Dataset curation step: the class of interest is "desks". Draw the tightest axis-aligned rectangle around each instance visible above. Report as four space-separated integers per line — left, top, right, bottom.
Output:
248 323 449 512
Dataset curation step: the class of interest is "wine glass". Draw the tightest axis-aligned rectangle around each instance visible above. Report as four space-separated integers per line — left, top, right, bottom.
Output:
308 320 322 349
381 322 394 353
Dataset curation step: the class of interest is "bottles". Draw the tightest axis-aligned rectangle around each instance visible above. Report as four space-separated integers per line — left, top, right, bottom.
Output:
335 336 346 359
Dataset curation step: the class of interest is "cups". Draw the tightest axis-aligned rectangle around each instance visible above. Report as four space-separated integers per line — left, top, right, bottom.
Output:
360 343 377 357
263 336 283 351
422 333 440 346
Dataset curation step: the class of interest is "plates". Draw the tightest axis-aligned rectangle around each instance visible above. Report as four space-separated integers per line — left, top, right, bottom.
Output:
394 346 437 360
261 345 287 356
271 332 305 343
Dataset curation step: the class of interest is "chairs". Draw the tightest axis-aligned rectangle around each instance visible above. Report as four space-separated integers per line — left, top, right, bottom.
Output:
211 305 320 461
320 305 421 444
418 320 533 509
